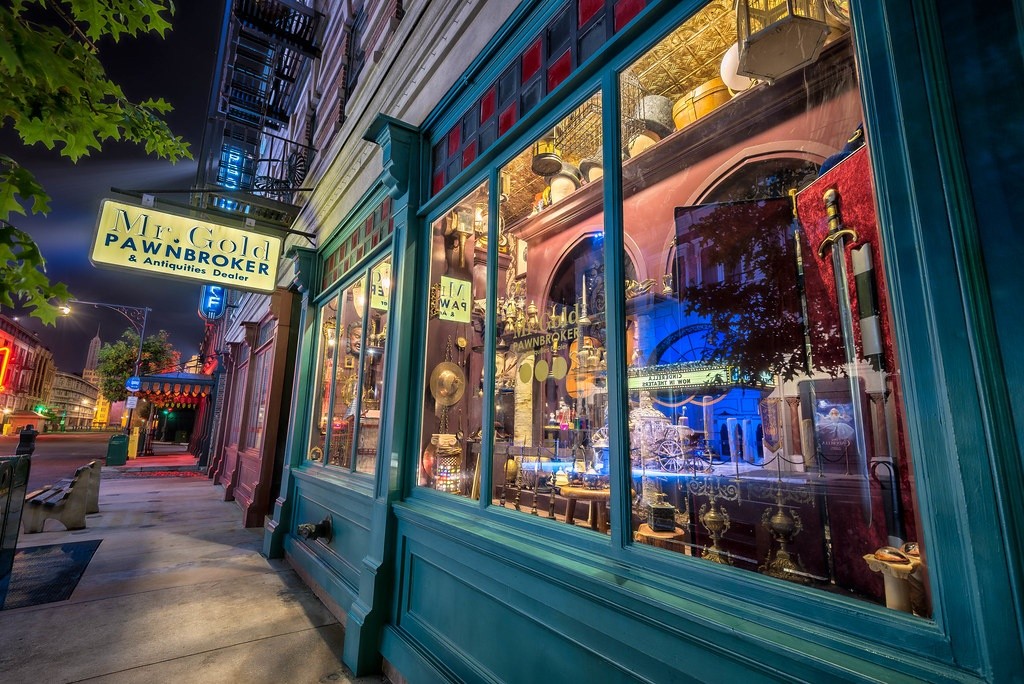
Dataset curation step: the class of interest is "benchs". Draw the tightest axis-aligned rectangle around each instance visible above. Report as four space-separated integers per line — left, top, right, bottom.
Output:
20 458 102 533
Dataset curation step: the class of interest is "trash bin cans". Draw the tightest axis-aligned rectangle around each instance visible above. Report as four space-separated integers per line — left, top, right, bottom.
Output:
106 434 129 466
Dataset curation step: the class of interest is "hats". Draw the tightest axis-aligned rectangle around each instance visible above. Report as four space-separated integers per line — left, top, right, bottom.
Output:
430 362 466 407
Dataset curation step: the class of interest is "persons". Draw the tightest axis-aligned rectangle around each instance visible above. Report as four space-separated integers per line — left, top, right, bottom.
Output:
346 320 363 354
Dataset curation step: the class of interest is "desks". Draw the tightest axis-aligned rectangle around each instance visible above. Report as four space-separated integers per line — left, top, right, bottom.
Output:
638 522 685 556
560 485 637 534
864 551 920 616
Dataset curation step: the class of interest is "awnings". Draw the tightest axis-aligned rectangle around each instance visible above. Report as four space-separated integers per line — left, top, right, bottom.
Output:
134 369 216 409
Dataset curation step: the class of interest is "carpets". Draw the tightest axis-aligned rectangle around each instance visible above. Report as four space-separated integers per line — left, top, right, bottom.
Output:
2 538 104 611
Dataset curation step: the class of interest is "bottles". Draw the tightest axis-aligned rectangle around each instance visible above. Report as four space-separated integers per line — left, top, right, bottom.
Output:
549 396 587 432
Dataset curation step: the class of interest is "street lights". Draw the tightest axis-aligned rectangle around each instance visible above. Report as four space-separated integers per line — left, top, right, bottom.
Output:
60 297 151 450
